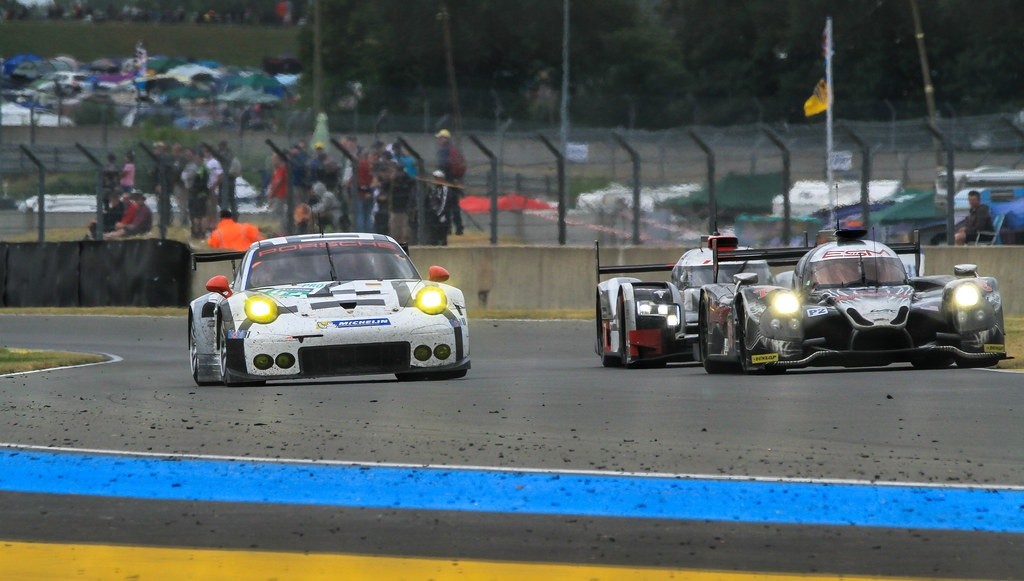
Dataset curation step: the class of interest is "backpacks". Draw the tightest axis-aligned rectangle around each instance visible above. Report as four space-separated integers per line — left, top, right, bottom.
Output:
224 150 241 176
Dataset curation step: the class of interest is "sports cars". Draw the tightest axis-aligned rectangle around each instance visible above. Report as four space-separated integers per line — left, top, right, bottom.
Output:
189 232 471 387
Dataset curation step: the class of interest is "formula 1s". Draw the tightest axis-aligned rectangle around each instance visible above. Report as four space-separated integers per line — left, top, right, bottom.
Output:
594 184 1016 376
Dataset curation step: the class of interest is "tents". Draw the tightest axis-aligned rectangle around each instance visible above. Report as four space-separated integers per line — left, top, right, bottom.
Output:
2 50 300 128
660 168 793 221
863 184 949 223
770 173 903 220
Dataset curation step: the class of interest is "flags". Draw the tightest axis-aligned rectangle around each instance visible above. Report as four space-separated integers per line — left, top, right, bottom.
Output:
804 77 831 116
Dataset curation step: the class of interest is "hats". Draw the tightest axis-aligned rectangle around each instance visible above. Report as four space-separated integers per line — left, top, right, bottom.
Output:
434 129 451 139
129 190 147 202
315 143 323 149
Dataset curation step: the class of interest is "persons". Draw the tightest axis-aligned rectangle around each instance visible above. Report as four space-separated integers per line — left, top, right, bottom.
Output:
954 191 995 244
429 167 454 244
434 128 467 235
91 136 417 241
312 183 344 231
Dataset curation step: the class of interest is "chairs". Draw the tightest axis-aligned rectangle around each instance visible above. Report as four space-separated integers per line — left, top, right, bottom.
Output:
968 213 1006 246
